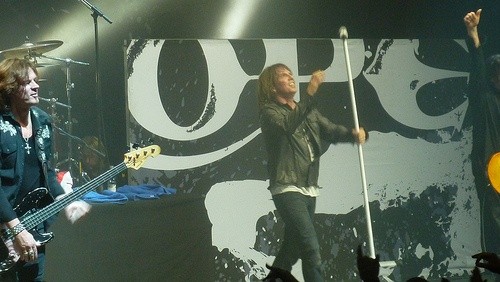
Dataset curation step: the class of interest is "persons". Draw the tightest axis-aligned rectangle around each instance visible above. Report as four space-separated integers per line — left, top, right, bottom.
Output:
0 57 92 282
255 63 370 282
80 135 110 188
463 9 500 282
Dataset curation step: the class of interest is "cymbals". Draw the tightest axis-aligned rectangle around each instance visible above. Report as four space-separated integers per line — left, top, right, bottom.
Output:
0 39 64 60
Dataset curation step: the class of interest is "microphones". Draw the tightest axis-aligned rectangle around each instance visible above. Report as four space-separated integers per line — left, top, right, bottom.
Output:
339 25 348 39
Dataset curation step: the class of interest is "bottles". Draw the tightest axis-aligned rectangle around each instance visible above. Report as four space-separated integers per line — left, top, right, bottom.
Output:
107 166 116 192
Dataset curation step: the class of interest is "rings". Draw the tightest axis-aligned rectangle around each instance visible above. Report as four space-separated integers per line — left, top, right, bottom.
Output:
29 251 34 256
24 252 28 256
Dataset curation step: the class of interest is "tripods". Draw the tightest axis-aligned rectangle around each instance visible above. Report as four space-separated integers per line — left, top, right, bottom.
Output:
31 51 91 185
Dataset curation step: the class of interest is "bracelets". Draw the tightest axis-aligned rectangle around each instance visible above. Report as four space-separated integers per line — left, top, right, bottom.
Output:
11 223 25 236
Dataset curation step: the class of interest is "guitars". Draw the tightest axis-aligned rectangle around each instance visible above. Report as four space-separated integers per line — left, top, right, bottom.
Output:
0 137 162 272
